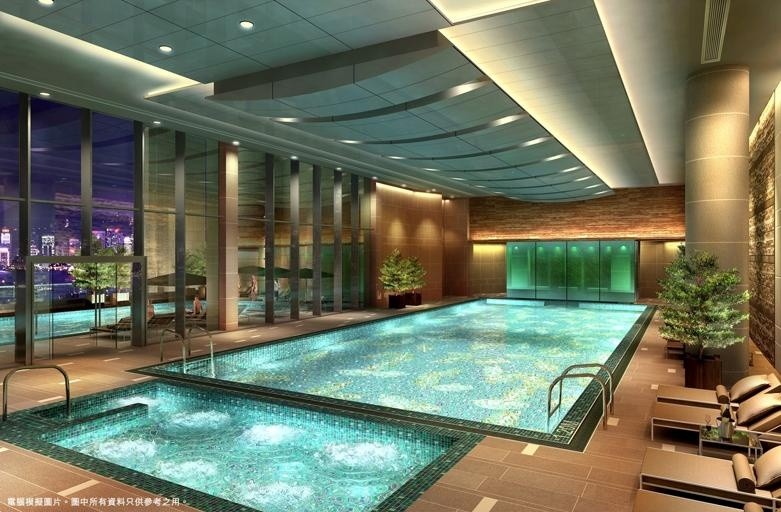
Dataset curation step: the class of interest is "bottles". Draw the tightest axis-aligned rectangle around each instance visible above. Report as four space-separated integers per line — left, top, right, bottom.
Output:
717 408 736 440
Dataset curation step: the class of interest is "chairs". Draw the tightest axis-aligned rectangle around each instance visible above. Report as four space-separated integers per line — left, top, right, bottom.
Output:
91 305 249 342
187 288 195 299
666 340 720 359
263 288 290 307
631 372 781 512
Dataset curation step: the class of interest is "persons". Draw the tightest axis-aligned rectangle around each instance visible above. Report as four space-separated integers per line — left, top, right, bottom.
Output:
237 274 328 312
185 296 203 316
146 300 155 321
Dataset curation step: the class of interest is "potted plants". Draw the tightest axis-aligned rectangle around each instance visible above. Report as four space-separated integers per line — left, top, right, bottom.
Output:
377 248 409 309
406 256 431 305
173 248 208 300
654 244 757 393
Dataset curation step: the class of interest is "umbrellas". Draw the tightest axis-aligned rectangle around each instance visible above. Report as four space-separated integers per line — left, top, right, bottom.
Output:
147 271 206 287
278 267 334 303
254 267 289 277
239 266 264 274
